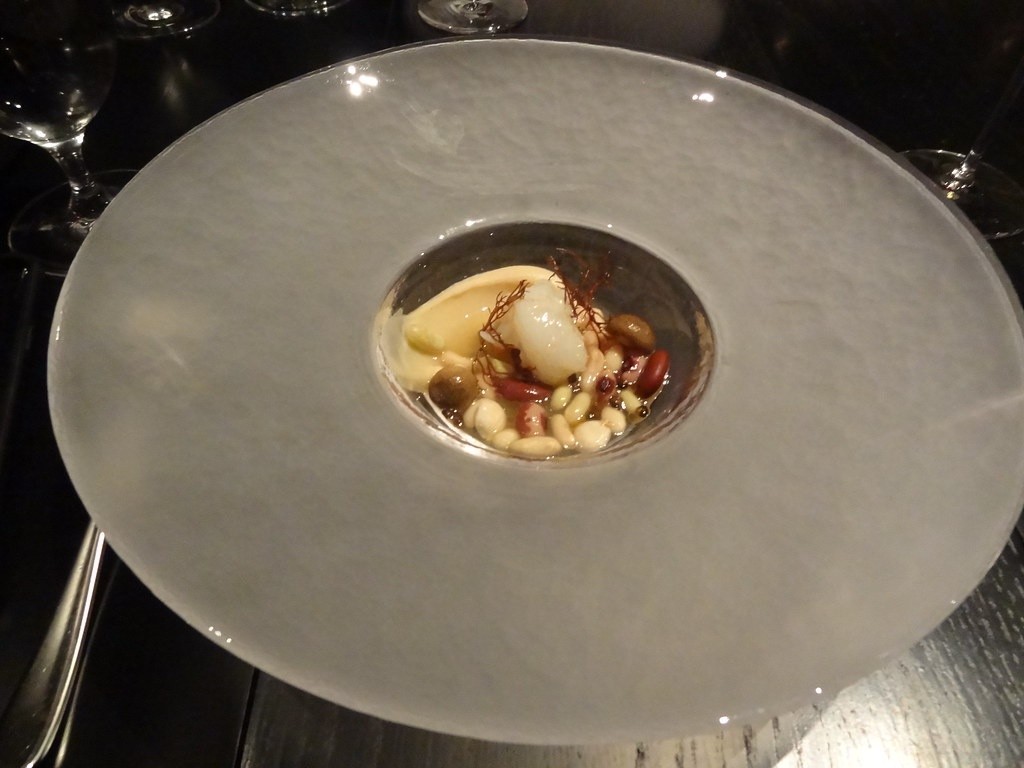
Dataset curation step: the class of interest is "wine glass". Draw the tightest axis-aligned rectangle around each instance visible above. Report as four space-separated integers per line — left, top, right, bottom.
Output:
0 0 138 276
898 59 1024 240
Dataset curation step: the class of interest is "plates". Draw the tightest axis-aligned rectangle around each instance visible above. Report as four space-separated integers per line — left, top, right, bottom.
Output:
48 40 1024 744
